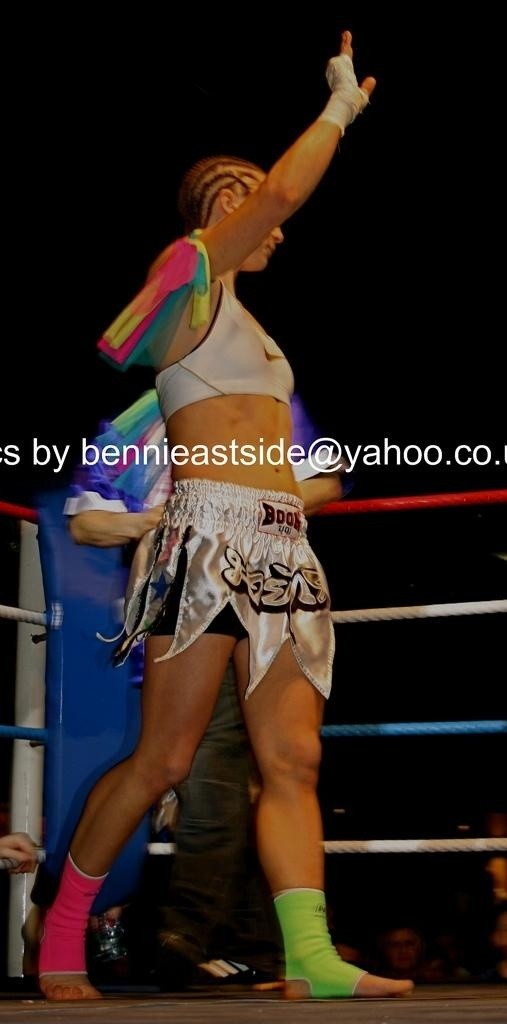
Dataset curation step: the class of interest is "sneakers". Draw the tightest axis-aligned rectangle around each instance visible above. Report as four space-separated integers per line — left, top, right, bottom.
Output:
173 958 285 994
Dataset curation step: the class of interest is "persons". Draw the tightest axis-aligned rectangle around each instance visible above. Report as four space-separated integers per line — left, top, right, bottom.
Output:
0 794 506 987
33 27 415 1003
60 385 348 993
